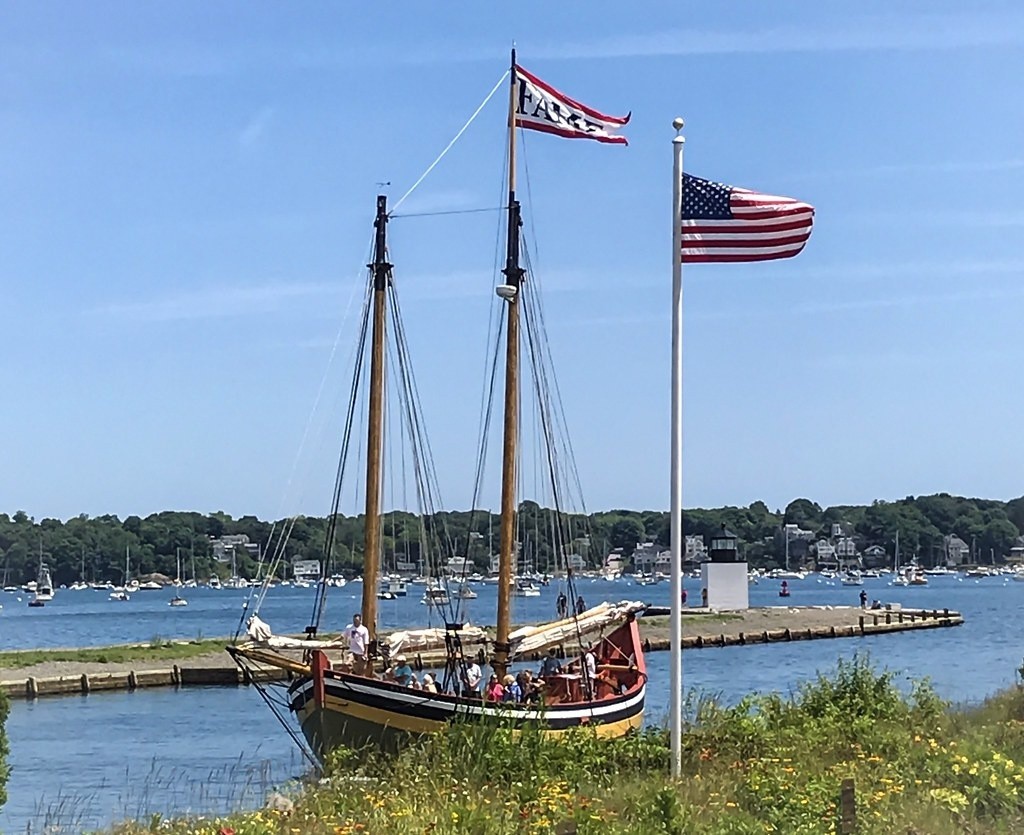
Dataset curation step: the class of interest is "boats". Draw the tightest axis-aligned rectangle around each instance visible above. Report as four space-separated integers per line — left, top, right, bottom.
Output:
0 526 1024 609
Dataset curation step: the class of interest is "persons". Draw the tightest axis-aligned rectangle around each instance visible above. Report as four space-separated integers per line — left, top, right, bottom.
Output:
556 591 567 619
485 643 596 705
860 590 881 610
332 614 370 677
460 654 482 699
386 655 441 695
681 588 707 608
576 596 587 615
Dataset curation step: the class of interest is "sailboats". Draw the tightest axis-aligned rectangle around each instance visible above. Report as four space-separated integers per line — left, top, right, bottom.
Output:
224 43 702 760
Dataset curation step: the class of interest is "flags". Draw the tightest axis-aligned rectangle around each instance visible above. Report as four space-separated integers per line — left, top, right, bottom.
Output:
680 171 816 263
511 65 633 146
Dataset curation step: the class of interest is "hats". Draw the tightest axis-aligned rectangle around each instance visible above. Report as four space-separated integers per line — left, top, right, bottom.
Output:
393 655 406 662
467 654 475 658
581 643 591 650
